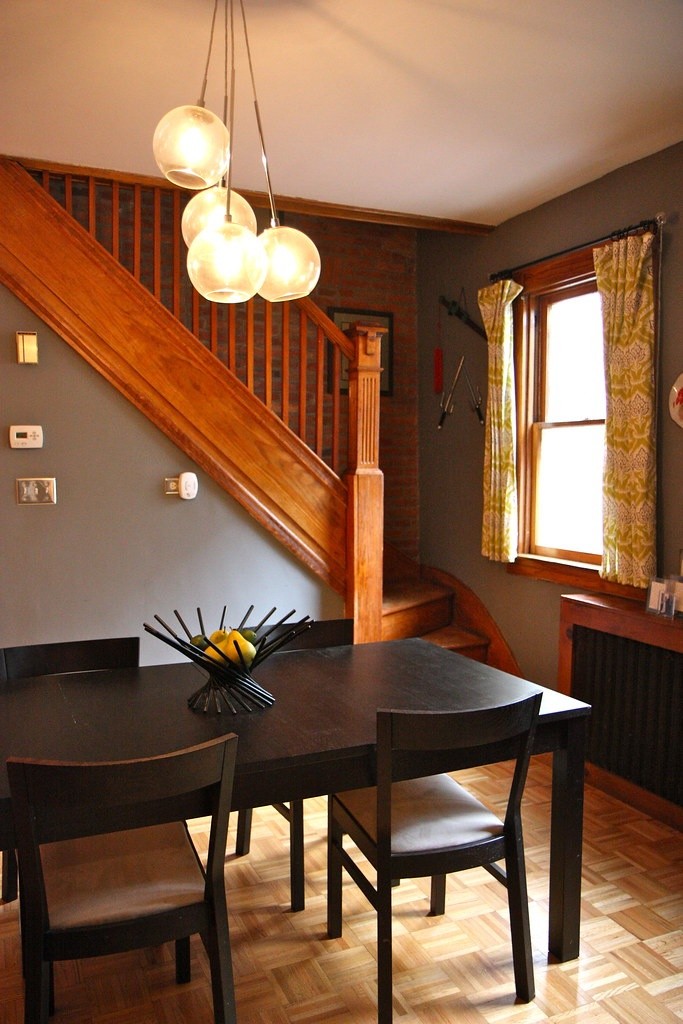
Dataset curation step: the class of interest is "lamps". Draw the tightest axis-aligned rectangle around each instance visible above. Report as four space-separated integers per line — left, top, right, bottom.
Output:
150 0 322 304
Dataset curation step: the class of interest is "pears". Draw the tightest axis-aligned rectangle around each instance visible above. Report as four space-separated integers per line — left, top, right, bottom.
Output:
190 625 258 666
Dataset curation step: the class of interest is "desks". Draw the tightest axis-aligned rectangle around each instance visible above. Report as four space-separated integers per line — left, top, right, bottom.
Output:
0 638 593 965
557 593 683 831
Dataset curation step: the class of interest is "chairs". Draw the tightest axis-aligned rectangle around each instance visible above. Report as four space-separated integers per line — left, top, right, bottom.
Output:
0 637 142 903
234 618 400 912
327 689 544 1024
5 732 241 1024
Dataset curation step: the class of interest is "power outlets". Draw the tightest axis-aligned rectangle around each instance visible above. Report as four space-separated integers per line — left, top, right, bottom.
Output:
164 477 179 495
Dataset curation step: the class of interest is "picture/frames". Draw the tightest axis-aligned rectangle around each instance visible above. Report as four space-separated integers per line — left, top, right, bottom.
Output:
15 477 57 505
326 307 394 397
